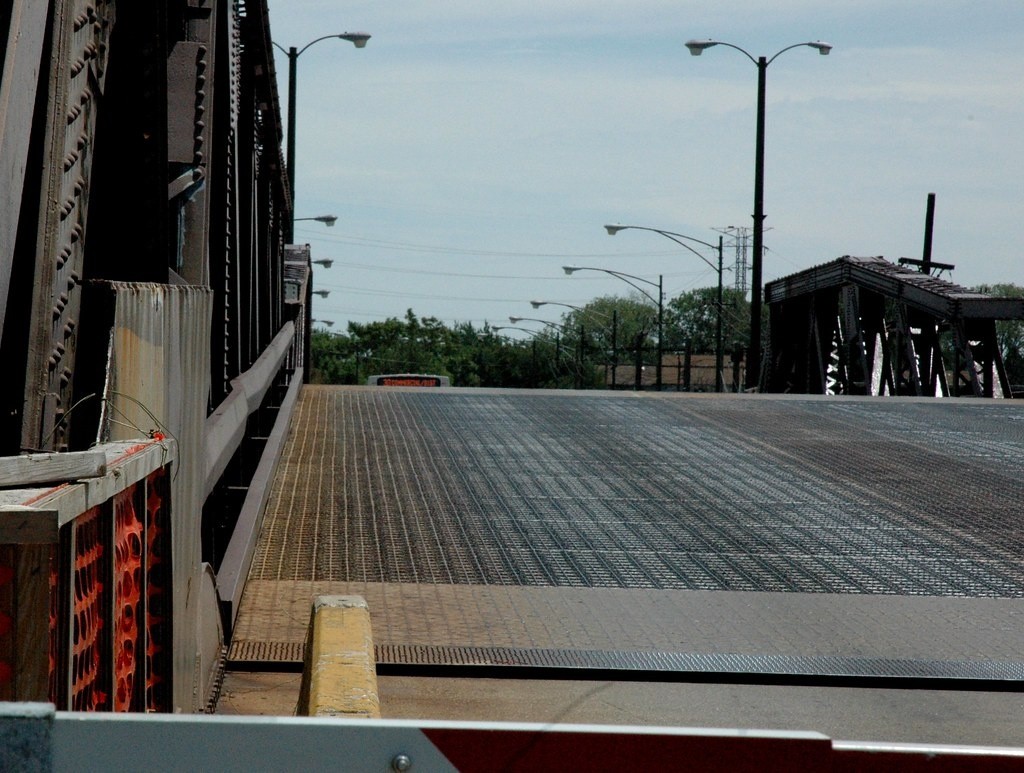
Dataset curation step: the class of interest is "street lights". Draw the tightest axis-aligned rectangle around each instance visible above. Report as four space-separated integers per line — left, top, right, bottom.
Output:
530 299 617 391
508 315 585 390
604 223 723 392
491 325 559 391
272 31 373 244
685 37 834 394
476 332 536 388
562 264 663 391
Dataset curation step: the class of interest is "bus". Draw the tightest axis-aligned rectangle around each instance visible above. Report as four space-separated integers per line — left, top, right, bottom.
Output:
368 373 450 386
